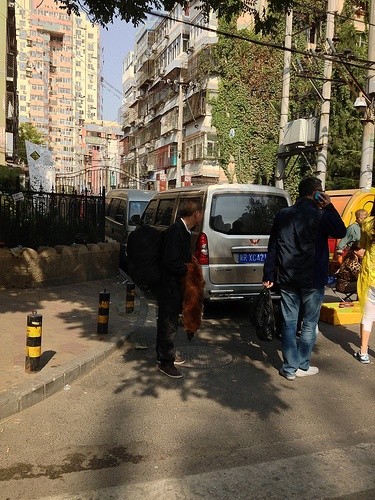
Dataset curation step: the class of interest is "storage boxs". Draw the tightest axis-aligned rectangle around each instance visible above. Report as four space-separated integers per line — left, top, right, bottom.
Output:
320 301 363 325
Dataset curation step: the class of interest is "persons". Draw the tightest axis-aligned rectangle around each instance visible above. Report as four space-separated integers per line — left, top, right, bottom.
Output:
261 176 348 380
157 199 204 379
335 197 375 363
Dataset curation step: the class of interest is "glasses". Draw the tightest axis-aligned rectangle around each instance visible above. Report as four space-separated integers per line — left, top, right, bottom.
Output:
319 190 325 193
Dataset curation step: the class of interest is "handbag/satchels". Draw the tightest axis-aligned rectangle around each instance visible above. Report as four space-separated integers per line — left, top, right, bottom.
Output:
252 285 276 341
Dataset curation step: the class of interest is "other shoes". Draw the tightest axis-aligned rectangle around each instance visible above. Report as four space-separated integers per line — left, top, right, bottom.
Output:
295 366 319 377
279 367 295 380
159 366 183 379
157 356 186 364
354 350 369 364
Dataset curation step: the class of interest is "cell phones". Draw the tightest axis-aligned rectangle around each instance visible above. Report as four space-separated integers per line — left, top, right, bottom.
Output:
315 192 324 201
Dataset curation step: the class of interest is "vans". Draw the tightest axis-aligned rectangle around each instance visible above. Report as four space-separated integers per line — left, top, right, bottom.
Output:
128 184 294 305
103 189 157 250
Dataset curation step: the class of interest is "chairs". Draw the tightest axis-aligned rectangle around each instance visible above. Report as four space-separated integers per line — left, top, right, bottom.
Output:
326 276 356 302
